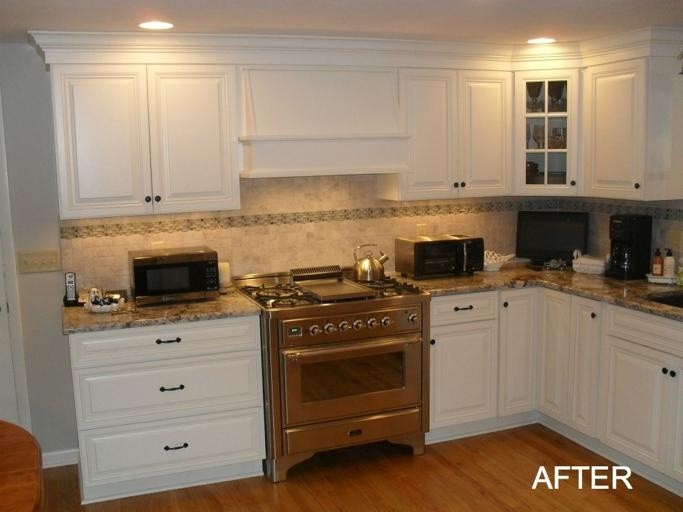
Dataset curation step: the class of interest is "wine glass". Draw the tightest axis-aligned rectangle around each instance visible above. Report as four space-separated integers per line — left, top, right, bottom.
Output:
525 82 566 150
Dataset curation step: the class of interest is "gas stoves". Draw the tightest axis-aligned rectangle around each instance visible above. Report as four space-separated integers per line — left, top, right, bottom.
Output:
236 273 429 353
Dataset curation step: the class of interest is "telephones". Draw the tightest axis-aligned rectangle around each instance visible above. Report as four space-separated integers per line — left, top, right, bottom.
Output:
63 272 78 305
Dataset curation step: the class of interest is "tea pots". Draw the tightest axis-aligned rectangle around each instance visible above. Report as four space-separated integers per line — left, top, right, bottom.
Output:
351 242 391 281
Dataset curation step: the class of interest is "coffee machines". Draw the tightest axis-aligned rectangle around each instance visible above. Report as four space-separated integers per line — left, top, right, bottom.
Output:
605 212 653 281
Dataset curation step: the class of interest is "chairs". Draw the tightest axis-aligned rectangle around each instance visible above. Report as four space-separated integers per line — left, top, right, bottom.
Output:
0 419 46 512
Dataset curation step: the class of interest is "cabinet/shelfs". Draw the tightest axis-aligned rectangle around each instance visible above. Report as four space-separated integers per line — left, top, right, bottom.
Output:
69 315 268 506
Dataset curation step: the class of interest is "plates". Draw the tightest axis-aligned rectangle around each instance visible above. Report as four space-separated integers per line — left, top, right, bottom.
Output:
643 272 675 285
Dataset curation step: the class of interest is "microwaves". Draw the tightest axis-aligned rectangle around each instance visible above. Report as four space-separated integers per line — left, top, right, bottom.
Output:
126 244 221 307
394 231 484 279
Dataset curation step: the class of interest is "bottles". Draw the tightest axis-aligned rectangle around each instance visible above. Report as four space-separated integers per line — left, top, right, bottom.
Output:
650 247 673 278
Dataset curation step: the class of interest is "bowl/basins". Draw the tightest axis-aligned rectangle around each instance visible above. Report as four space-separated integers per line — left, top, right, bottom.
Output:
83 298 127 314
484 252 515 273
571 261 603 276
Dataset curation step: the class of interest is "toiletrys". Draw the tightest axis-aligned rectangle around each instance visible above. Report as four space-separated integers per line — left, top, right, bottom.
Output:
664 248 675 278
653 248 663 275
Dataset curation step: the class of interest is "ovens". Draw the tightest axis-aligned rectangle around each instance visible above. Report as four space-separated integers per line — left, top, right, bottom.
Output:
261 335 429 482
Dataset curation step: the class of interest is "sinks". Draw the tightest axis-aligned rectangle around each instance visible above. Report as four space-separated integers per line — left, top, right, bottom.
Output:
646 290 683 308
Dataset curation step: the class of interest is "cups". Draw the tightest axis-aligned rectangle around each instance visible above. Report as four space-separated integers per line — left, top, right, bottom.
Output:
525 161 538 183
217 261 234 294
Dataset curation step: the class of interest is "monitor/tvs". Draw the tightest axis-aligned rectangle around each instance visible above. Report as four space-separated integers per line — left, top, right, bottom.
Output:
516 211 588 270
